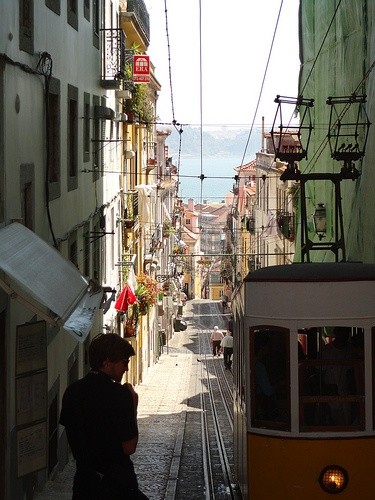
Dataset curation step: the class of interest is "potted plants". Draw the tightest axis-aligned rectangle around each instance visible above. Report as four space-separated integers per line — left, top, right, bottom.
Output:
126 83 154 132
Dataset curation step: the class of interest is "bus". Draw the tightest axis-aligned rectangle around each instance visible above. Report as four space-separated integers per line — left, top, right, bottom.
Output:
226 261 375 500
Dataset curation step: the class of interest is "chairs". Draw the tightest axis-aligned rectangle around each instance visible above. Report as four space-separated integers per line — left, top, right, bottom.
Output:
299 359 365 431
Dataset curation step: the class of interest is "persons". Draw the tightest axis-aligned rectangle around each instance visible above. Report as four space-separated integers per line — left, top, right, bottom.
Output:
226 315 233 337
210 326 225 356
60 336 151 500
220 331 234 370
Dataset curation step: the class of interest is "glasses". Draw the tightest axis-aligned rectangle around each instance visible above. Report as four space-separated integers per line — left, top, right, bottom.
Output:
120 356 130 364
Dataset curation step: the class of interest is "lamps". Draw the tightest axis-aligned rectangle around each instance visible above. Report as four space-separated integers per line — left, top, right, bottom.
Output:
313 203 326 241
